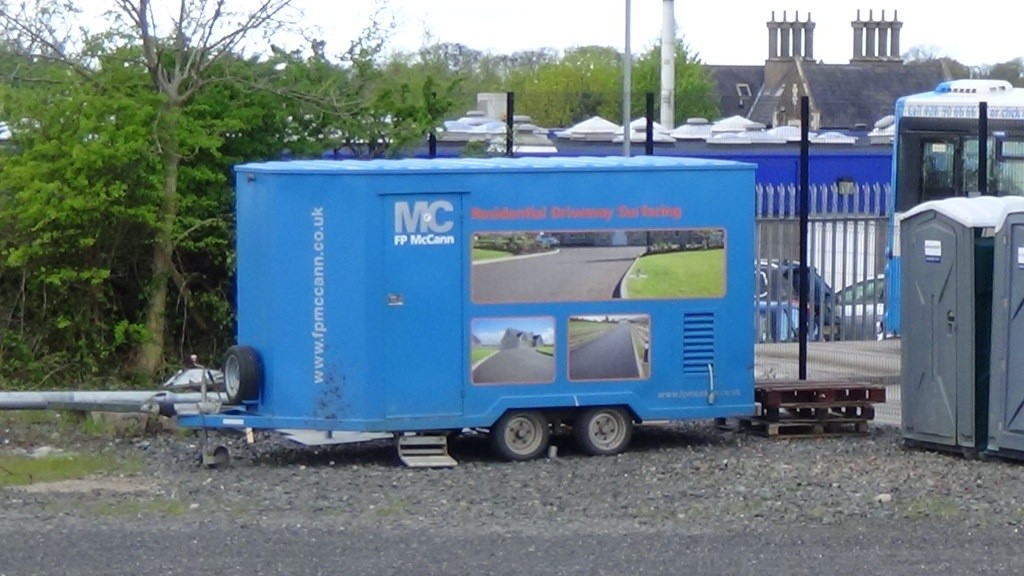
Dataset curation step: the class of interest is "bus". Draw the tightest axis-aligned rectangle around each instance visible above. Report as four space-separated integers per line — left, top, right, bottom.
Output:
881 80 1024 334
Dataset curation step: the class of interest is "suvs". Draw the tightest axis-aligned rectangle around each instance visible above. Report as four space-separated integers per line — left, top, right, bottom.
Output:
754 258 843 343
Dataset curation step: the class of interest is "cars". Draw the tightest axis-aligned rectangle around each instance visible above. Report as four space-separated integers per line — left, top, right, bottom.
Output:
536 234 560 247
827 274 884 340
